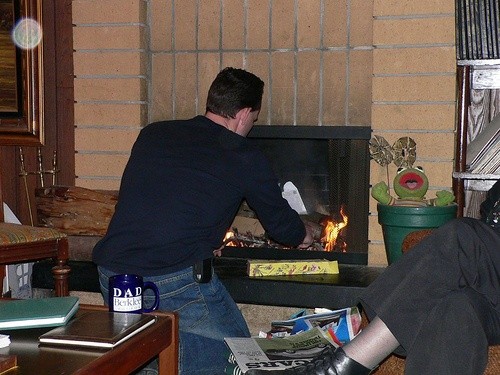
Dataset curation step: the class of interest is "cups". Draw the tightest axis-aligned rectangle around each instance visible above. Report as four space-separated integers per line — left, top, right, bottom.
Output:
109 274 160 314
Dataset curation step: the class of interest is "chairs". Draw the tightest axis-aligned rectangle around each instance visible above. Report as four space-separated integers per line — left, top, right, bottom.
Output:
0 176 70 298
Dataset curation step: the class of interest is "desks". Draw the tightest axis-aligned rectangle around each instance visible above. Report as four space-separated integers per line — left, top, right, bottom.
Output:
0 303 178 375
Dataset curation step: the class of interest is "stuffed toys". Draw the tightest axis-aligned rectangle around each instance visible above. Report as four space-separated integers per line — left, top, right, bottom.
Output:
371 166 457 206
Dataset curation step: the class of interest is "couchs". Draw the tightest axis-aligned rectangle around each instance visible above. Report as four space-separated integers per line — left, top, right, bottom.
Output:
360 228 500 375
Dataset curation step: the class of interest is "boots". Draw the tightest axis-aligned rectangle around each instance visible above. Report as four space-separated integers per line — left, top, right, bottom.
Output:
245 346 373 375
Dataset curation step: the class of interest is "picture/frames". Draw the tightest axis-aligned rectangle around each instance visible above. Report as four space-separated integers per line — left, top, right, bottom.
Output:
0 0 47 147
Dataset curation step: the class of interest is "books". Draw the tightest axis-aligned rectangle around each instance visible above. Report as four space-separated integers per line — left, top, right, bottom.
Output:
465 114 500 176
1 295 82 331
39 310 157 350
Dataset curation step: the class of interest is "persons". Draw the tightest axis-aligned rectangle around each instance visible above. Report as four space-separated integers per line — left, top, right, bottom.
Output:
243 177 500 375
91 65 314 375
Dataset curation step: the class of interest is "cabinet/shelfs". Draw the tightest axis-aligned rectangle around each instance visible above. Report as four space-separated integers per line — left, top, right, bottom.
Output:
453 0 500 228
